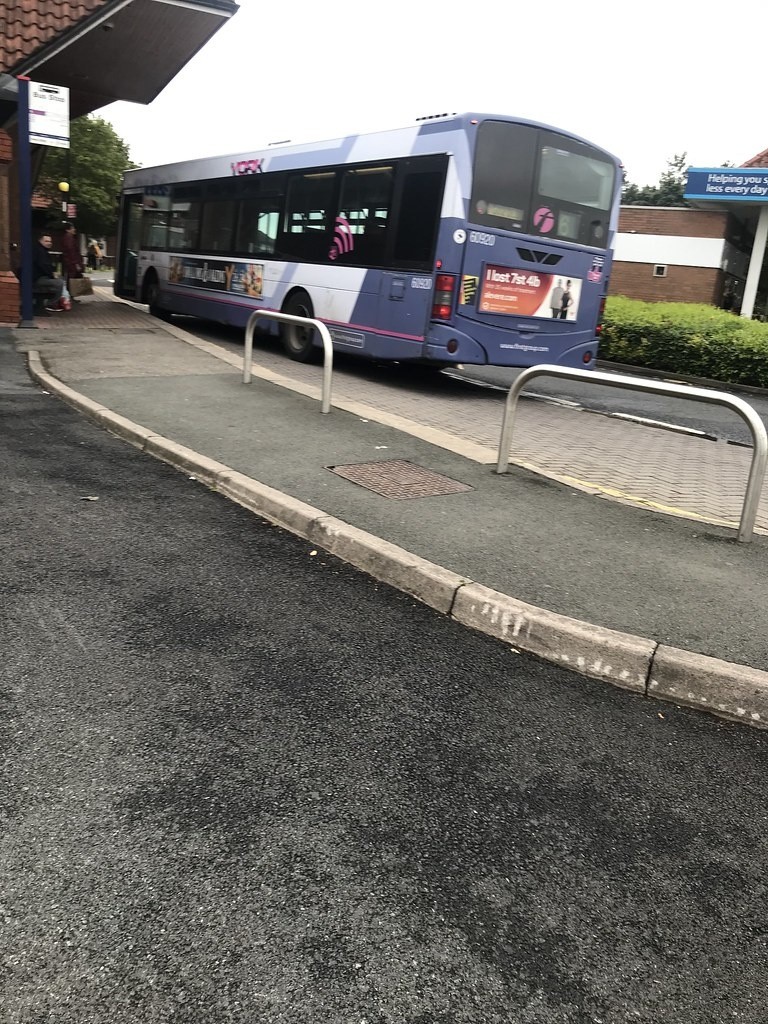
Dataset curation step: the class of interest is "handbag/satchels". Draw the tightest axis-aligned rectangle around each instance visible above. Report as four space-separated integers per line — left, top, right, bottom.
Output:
69 272 94 297
60 284 72 311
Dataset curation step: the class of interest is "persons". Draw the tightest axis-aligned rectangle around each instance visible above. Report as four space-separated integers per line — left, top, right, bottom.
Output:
86 245 99 271
61 223 84 304
550 278 574 319
30 232 65 311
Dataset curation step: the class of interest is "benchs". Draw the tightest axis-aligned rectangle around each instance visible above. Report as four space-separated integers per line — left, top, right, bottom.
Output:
17 267 57 318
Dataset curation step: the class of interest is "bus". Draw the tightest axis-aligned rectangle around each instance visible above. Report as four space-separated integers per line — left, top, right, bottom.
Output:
112 108 624 377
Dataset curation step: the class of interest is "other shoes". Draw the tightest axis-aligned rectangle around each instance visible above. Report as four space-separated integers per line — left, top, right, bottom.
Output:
44 304 64 311
